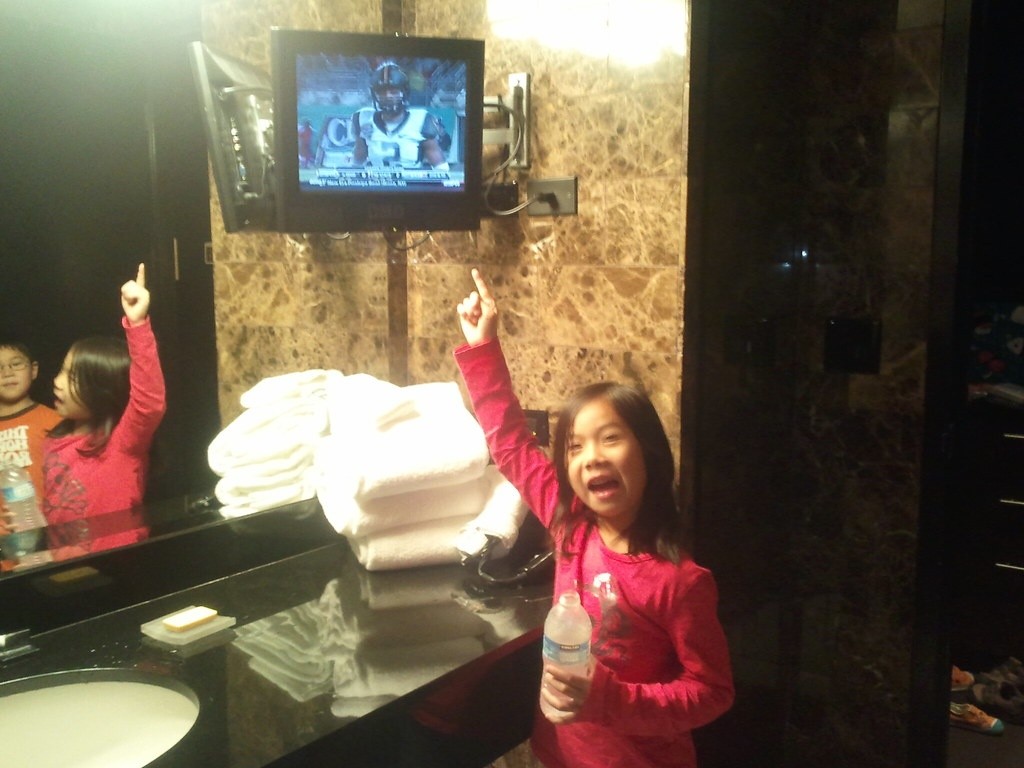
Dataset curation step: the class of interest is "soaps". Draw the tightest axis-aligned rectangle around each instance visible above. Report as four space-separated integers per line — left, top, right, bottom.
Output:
162 606 218 632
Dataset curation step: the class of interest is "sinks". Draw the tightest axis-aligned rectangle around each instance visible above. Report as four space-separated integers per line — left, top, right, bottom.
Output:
0 667 200 768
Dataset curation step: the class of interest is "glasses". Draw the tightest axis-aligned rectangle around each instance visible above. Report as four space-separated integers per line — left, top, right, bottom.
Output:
0 361 33 374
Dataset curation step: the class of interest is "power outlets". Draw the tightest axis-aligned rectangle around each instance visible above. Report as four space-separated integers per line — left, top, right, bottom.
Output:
524 409 549 446
525 176 578 216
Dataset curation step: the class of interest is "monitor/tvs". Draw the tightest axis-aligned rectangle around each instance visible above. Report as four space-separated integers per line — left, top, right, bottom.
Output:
270 27 486 234
186 41 276 234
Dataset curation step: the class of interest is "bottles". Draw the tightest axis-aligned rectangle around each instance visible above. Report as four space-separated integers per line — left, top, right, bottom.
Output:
0 459 49 531
538 589 593 721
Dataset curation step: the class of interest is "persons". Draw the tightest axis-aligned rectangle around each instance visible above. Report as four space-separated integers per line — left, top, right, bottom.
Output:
297 61 460 187
0 334 60 535
451 266 735 767
41 263 169 525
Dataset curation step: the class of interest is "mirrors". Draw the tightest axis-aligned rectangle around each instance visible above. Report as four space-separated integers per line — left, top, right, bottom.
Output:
0 0 405 581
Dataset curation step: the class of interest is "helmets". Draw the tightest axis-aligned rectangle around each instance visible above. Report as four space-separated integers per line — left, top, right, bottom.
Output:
370 62 407 115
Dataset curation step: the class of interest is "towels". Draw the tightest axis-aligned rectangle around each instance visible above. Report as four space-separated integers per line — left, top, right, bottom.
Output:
316 378 529 572
205 369 345 519
231 552 494 718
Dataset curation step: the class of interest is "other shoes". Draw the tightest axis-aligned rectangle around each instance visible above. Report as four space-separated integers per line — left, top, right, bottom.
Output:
949 657 1024 734
